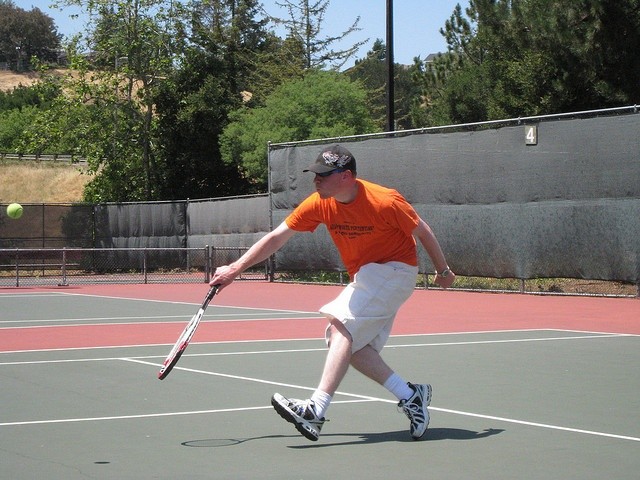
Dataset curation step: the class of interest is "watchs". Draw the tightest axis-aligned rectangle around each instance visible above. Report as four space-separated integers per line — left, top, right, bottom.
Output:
434 265 450 279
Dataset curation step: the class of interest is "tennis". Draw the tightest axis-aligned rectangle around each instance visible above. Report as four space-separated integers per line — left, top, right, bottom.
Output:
7 203 22 218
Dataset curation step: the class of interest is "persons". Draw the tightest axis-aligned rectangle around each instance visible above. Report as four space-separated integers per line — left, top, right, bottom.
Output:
208 144 455 441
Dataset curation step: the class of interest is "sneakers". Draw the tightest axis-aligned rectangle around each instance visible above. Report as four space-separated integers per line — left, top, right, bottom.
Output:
396 380 432 441
270 391 332 442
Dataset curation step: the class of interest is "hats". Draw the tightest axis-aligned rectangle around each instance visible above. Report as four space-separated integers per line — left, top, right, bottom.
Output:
301 143 359 179
